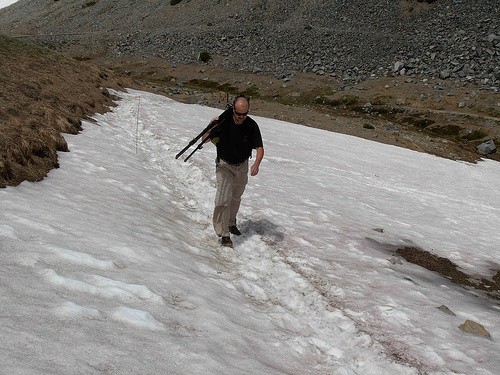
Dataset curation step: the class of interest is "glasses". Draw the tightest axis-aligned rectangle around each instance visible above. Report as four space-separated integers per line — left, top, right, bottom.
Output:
235 111 248 116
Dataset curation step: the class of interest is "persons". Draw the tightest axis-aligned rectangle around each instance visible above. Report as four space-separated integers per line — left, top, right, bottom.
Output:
202 96 264 247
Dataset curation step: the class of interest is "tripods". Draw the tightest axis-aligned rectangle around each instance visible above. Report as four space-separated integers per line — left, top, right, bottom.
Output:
176 104 234 162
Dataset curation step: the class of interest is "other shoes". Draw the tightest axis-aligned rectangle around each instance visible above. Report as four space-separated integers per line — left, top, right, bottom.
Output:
222 236 233 246
229 226 241 235
